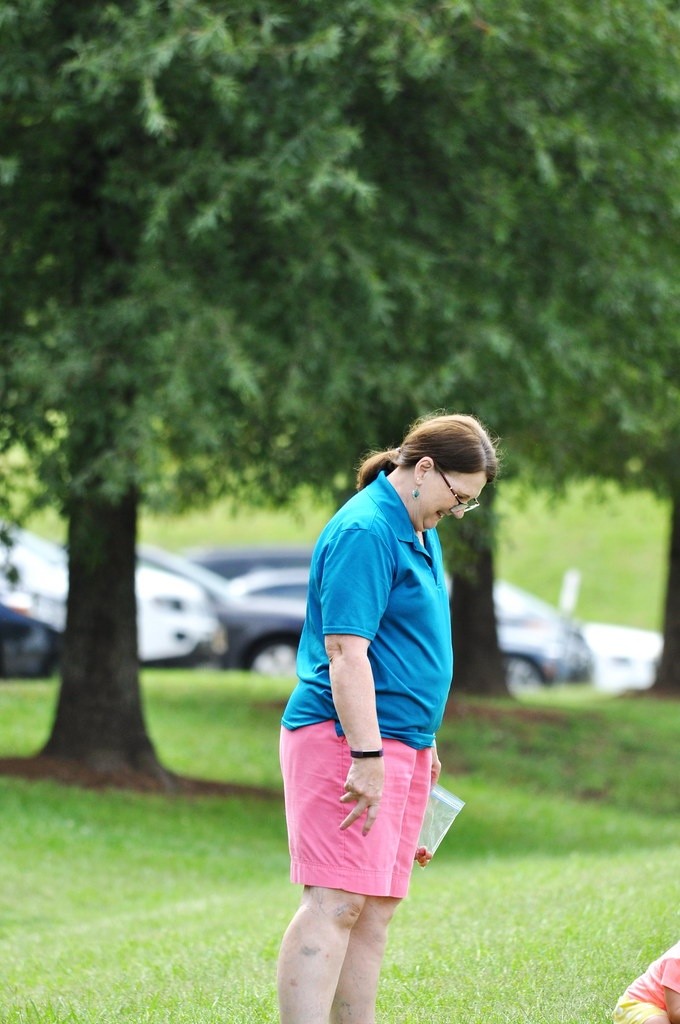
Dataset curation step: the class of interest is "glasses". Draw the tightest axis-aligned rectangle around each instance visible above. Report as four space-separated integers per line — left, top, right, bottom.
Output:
436 464 480 513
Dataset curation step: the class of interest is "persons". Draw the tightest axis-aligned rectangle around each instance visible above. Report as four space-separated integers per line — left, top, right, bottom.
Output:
278 413 500 1023
613 938 680 1024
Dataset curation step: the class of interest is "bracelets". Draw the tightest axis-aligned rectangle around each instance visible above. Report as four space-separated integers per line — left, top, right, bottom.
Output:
350 747 385 758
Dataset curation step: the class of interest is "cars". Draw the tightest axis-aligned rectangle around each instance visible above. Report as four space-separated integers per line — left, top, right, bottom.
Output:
0 529 312 677
234 567 594 689
